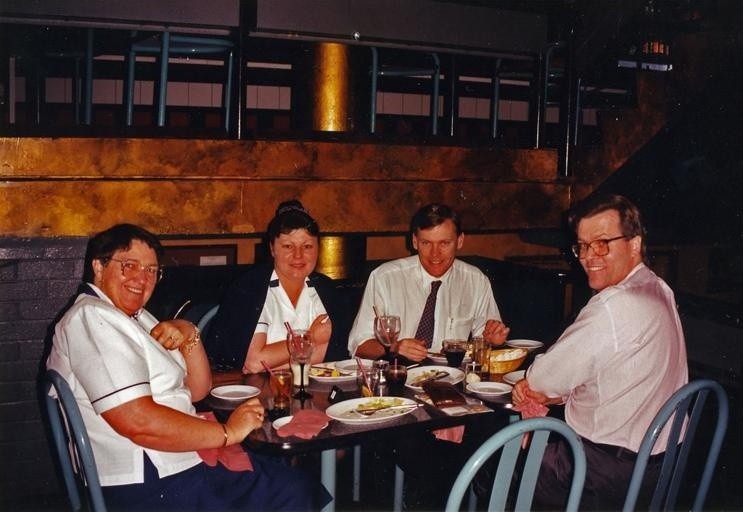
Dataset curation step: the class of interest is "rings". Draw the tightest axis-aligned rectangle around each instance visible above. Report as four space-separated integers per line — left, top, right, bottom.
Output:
170 335 176 343
256 412 261 420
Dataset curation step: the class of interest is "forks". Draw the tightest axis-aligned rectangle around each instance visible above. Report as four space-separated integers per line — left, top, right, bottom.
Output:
352 403 424 417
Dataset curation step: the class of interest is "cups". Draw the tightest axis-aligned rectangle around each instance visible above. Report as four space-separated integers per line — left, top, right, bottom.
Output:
355 360 406 400
471 337 491 378
442 339 466 366
268 371 292 409
289 354 310 388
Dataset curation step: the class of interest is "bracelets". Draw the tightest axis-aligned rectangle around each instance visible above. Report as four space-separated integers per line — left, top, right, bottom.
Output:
220 423 229 448
181 323 201 357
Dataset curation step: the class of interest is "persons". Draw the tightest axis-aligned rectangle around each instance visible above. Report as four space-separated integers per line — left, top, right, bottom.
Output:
346 203 511 512
203 198 354 473
456 192 691 512
44 222 335 512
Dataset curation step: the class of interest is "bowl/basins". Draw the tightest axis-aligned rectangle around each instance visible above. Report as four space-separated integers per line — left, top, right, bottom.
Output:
488 348 529 374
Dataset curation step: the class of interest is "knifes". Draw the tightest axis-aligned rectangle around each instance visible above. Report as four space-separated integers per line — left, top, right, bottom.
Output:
411 372 448 387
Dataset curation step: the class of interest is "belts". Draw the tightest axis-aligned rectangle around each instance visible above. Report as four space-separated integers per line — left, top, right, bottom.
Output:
599 444 640 464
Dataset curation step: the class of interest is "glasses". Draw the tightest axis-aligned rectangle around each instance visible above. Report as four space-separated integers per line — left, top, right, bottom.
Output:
108 258 164 284
571 234 631 259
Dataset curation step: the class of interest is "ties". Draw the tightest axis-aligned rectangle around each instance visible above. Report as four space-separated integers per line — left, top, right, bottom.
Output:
415 282 440 348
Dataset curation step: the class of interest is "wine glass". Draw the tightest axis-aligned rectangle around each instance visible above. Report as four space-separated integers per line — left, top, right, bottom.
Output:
373 316 401 362
286 329 317 400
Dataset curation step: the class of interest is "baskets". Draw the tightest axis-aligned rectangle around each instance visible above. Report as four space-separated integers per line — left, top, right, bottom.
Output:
470 348 528 374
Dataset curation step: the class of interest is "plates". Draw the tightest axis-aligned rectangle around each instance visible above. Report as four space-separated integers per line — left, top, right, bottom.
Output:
307 358 376 383
402 365 466 393
209 384 261 402
272 415 328 431
465 382 513 398
426 353 471 364
502 370 527 385
505 339 543 353
326 396 419 426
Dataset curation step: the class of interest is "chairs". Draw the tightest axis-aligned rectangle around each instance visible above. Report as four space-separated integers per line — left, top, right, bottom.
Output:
33 360 112 511
440 377 732 512
